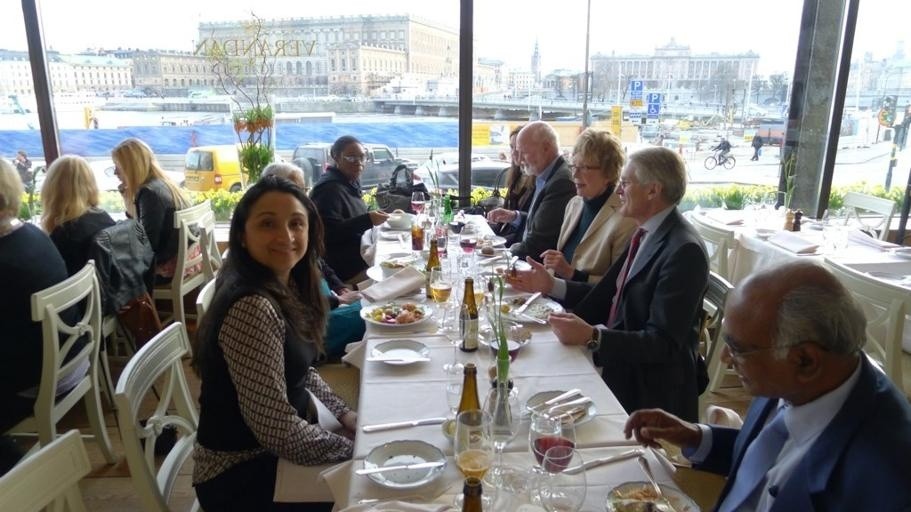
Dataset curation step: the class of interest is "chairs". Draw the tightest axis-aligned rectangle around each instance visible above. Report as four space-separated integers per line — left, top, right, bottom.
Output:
682 188 911 396
0 185 313 511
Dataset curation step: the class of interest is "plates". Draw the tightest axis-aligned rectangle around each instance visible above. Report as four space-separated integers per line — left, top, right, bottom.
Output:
492 293 567 324
374 339 429 368
382 231 409 239
482 258 532 273
364 439 445 490
383 252 417 263
605 479 702 511
477 326 531 349
363 262 407 282
477 234 508 247
359 299 432 326
383 222 411 231
524 392 598 425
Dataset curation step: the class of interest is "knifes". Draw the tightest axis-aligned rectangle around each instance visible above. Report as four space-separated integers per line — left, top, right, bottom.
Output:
513 292 542 316
364 357 428 363
354 459 446 479
361 419 447 433
559 447 645 474
518 386 579 416
483 255 505 266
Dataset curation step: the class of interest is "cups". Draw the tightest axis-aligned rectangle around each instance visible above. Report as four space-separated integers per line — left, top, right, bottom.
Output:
493 320 524 363
537 445 588 511
822 208 846 260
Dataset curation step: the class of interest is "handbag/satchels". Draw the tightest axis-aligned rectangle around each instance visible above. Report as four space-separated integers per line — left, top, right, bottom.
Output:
138 418 177 456
374 183 431 215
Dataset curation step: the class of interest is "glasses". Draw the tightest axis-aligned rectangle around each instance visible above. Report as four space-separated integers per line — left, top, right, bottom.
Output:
569 163 602 173
343 154 368 164
723 341 795 364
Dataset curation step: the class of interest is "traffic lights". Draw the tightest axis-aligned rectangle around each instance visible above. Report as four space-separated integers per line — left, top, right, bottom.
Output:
884 94 898 125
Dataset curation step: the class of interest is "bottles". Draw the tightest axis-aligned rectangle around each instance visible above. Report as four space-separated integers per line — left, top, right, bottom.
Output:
458 277 480 352
461 477 484 512
453 364 485 454
785 206 803 232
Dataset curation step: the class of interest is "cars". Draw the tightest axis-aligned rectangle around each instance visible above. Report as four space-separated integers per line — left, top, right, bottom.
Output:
745 103 765 116
409 149 571 198
88 84 163 98
86 162 187 190
184 142 305 194
636 119 719 143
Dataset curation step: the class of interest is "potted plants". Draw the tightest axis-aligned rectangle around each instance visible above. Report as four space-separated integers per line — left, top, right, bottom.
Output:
190 10 293 192
768 151 798 213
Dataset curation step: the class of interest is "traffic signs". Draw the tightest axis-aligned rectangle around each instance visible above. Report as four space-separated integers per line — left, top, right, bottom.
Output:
878 108 896 126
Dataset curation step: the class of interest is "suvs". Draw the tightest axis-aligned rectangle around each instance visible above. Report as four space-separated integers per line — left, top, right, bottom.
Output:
293 143 420 193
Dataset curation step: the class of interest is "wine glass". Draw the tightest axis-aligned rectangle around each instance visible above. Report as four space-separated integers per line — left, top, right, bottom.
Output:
411 192 482 333
451 409 494 509
441 363 466 439
441 303 473 372
479 387 526 490
529 411 578 505
749 184 779 217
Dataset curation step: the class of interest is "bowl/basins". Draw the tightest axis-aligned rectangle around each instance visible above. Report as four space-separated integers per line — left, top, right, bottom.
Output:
386 214 413 226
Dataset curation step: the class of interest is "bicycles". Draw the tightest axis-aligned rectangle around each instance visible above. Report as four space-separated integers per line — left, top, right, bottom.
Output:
705 146 737 170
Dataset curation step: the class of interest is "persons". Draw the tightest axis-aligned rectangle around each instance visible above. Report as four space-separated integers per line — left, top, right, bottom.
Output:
40 156 117 358
779 132 785 159
483 123 712 425
12 150 34 193
711 137 731 166
190 134 390 512
750 132 764 161
624 263 911 512
898 105 910 152
111 140 203 358
1 158 91 476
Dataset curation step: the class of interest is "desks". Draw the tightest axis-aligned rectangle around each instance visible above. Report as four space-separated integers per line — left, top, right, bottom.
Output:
342 210 708 512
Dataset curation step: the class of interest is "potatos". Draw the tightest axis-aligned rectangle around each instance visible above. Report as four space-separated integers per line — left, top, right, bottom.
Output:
497 302 509 312
481 246 494 253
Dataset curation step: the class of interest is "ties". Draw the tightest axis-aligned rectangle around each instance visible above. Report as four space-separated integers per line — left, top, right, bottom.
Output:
718 407 792 512
606 227 648 329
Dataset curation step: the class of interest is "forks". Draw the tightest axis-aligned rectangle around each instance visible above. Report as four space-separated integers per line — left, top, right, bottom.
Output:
527 397 592 419
357 483 452 503
639 456 676 511
